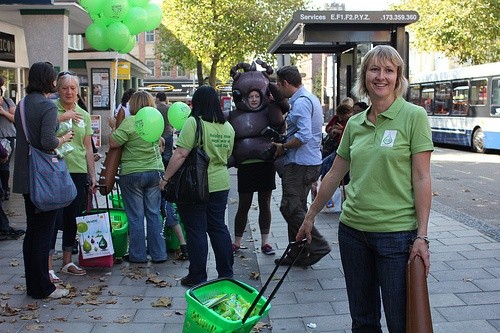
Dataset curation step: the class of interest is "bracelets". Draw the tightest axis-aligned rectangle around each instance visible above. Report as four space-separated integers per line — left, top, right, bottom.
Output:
163 175 169 182
412 236 429 248
62 136 66 142
282 144 289 151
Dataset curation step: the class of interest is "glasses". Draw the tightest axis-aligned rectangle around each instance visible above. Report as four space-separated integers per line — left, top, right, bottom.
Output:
57 71 77 84
46 61 53 69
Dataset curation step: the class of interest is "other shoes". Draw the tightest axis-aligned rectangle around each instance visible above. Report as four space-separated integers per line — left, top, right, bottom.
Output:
48 288 69 298
2 191 9 200
302 250 331 268
172 251 179 260
124 255 146 263
181 274 207 286
274 251 307 268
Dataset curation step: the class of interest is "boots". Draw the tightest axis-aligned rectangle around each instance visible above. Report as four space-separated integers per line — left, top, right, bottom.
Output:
179 244 190 259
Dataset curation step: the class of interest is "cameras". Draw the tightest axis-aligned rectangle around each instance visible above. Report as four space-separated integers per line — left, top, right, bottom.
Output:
260 127 286 159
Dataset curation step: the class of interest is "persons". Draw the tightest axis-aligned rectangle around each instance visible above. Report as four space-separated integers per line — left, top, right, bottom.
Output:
159 86 235 287
226 58 290 254
311 98 370 202
49 69 97 283
108 89 189 265
272 66 331 270
0 77 25 240
296 45 434 333
12 61 73 299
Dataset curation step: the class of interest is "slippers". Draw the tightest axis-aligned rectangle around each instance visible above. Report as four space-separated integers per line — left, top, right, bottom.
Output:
60 263 87 276
49 269 60 283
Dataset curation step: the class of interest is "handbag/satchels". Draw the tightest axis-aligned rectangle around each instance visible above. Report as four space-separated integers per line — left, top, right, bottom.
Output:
29 144 78 213
321 134 333 147
0 138 14 163
317 175 343 213
76 188 115 267
166 115 210 203
313 181 317 199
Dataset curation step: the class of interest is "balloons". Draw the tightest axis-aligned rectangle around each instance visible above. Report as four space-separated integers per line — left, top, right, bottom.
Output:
167 102 191 129
135 107 164 142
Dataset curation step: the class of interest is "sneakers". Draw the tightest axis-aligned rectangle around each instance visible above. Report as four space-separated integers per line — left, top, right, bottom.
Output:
261 244 275 255
232 243 240 252
0 226 25 240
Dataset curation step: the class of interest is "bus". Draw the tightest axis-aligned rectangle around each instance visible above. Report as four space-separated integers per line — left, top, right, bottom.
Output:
406 62 500 154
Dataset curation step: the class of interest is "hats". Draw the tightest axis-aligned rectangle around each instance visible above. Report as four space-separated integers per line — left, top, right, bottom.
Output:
354 102 369 110
341 97 354 107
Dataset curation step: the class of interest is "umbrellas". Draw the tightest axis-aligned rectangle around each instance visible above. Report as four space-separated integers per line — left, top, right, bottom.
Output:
78 0 162 54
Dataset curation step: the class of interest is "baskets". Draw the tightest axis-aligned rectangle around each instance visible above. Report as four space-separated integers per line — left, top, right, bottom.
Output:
83 208 129 262
181 277 272 333
109 194 126 214
